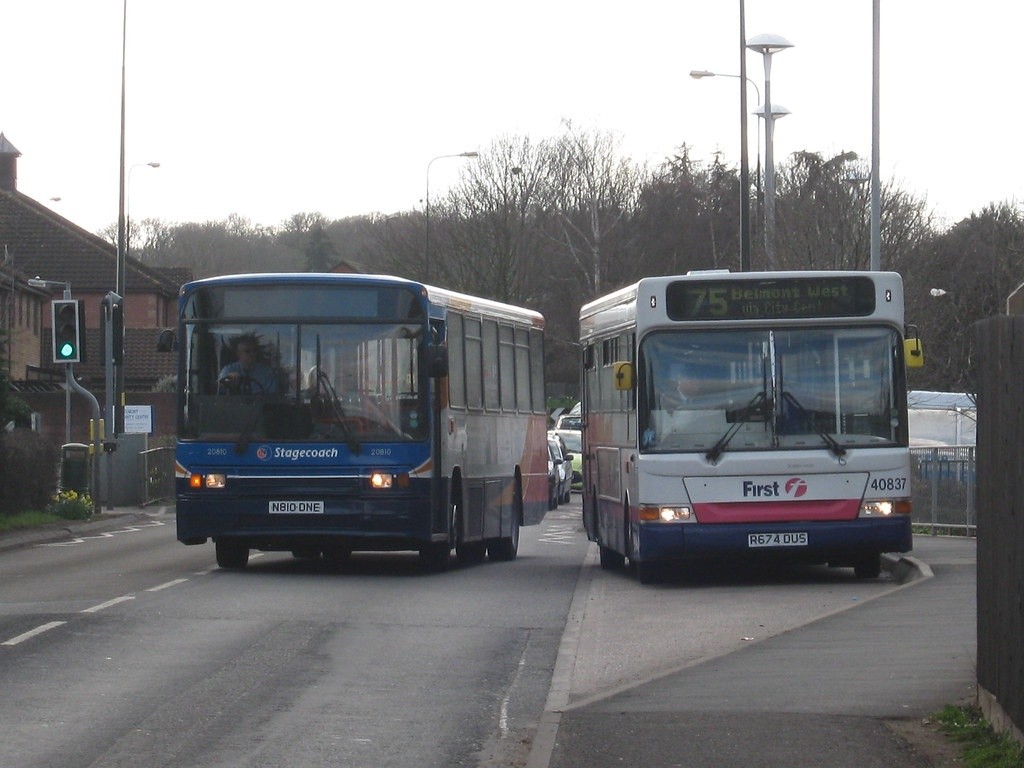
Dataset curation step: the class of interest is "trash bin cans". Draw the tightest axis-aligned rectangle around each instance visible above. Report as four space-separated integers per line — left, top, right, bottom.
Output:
59 443 90 497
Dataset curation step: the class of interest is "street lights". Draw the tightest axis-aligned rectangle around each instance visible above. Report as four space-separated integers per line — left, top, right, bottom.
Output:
747 31 794 271
689 68 761 272
124 161 161 254
424 150 479 281
26 278 72 458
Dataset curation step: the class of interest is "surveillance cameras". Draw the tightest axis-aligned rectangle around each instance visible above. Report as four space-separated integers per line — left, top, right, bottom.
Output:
107 290 124 305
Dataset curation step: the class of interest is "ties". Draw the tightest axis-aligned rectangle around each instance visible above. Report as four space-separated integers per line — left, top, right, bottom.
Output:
242 368 251 394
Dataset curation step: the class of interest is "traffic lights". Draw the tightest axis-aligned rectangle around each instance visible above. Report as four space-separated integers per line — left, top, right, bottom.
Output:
50 299 81 363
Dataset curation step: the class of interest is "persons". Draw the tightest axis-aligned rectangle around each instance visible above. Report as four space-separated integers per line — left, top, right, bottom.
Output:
301 365 317 399
644 367 736 448
219 339 276 411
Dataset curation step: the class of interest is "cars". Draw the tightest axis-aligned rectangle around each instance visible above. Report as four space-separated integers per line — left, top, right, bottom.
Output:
547 399 582 509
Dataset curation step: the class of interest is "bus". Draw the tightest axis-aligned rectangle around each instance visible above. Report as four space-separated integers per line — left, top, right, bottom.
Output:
155 271 554 575
577 267 926 583
